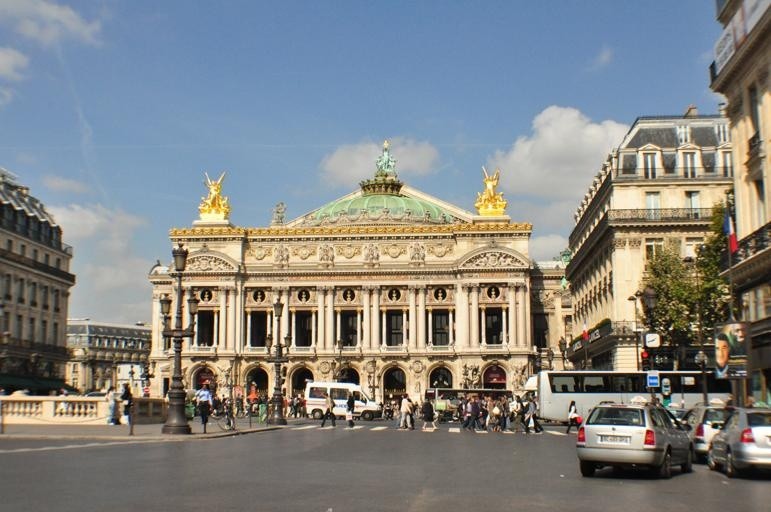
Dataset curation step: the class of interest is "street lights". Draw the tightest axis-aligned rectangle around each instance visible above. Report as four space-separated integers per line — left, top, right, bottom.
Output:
163 245 201 436
139 360 156 400
264 292 293 426
331 339 353 384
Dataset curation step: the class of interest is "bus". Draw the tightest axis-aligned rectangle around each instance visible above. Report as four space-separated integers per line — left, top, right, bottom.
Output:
537 368 734 424
424 386 514 422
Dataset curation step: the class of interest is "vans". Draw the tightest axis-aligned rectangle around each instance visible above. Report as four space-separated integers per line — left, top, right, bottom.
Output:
304 378 382 421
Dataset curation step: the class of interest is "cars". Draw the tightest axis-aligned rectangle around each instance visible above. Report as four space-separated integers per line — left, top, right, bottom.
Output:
706 407 771 479
574 401 695 478
680 402 738 463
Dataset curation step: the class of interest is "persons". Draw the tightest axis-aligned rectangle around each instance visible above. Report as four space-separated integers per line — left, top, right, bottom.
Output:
715 333 732 378
116 383 133 425
104 386 121 426
727 324 750 356
2 382 74 412
566 401 583 436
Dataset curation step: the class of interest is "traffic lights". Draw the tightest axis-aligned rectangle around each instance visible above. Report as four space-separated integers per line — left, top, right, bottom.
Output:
640 351 650 371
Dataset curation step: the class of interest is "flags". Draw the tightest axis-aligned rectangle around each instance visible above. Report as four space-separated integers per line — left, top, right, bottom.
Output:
583 315 590 350
725 203 738 255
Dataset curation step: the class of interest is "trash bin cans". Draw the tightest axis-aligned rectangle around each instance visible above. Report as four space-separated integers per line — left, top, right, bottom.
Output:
184 405 195 420
259 404 268 424
